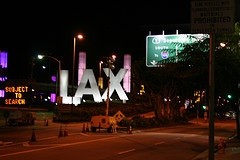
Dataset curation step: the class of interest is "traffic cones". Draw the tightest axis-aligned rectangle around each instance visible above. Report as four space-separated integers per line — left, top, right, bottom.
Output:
64 125 69 136
59 125 64 137
82 122 91 133
127 125 132 134
31 128 38 141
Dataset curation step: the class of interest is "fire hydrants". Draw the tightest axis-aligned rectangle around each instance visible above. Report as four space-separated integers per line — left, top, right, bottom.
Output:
217 138 226 154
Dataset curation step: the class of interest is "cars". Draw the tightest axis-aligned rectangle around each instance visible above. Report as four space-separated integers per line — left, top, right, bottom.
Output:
8 112 36 126
225 111 236 119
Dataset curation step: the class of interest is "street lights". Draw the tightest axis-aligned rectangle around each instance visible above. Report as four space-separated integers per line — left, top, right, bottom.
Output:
37 54 61 124
106 54 117 130
72 33 83 120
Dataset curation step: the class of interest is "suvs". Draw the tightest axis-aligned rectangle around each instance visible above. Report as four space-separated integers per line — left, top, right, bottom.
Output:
120 109 156 126
